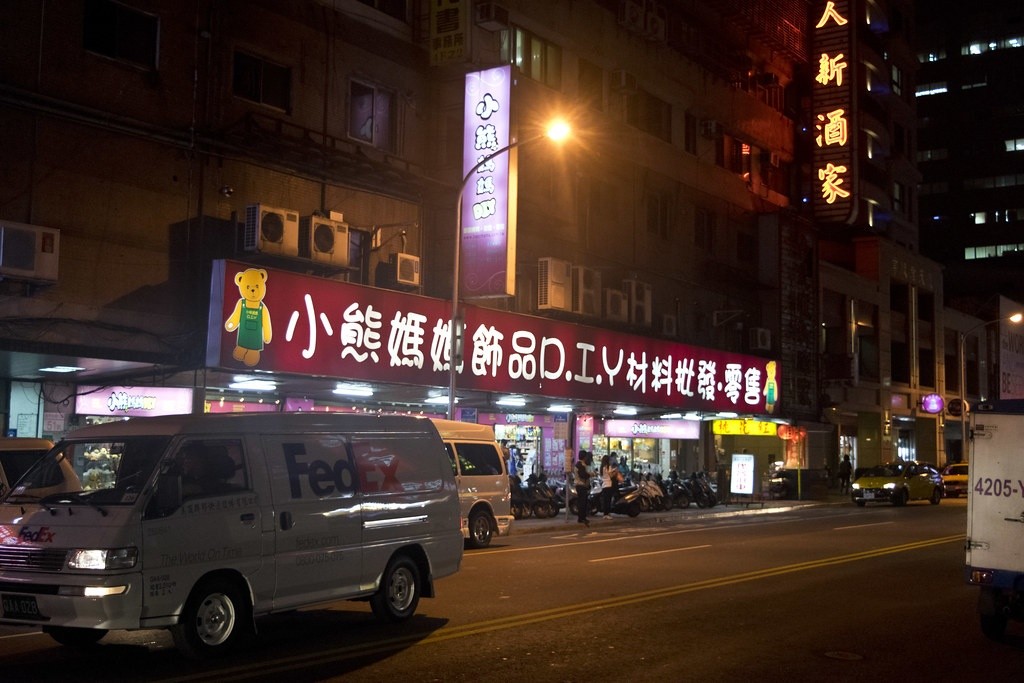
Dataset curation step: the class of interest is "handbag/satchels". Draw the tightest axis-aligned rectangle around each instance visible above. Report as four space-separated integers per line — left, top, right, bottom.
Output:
612 478 619 488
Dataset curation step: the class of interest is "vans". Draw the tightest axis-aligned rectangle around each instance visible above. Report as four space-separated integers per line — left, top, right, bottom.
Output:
429 419 515 548
1 409 464 664
0 436 85 503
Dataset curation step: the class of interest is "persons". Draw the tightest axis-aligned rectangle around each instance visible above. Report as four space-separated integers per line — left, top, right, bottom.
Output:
499 439 510 462
600 452 629 520
840 455 852 495
574 450 596 524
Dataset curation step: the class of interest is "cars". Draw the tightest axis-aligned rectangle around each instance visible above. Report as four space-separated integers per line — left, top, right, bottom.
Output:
941 463 968 498
850 460 945 508
765 461 785 494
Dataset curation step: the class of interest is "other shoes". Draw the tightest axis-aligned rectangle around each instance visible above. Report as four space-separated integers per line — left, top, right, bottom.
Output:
578 518 589 523
603 515 613 519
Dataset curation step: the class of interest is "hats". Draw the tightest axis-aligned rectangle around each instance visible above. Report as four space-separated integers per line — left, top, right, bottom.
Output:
611 452 618 456
621 456 627 460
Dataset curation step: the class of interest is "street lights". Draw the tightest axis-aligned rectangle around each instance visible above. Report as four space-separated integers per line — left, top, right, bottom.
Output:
447 117 573 421
960 312 1024 463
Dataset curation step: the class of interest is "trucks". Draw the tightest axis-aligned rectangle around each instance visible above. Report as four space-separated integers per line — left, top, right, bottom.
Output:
961 400 1024 640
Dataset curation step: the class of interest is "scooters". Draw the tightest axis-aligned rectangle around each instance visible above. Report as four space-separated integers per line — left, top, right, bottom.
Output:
508 455 720 521
